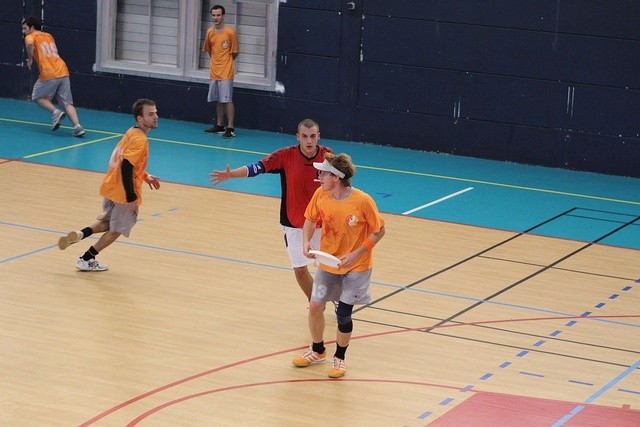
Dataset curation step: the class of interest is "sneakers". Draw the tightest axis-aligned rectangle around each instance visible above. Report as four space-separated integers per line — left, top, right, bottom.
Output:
223 130 235 139
51 109 66 130
329 356 346 377
72 125 85 136
204 125 226 133
59 231 83 250
292 346 326 367
76 257 108 271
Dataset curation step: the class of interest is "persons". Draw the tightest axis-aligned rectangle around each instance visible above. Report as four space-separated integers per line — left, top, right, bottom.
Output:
21 16 86 137
206 116 340 316
57 98 161 272
201 5 239 138
291 151 386 379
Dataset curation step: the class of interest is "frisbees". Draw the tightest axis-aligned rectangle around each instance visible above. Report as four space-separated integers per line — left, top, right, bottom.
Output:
309 249 341 268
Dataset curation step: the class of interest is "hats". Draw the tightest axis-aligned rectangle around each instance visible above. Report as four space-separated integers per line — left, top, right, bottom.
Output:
313 159 347 178
